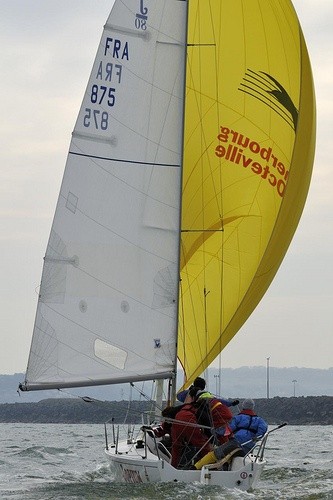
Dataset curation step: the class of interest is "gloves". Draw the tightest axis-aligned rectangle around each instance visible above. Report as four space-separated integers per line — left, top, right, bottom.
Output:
141 426 150 432
232 400 239 406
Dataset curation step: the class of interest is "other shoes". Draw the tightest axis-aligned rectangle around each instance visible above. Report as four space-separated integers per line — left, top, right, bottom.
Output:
188 465 197 470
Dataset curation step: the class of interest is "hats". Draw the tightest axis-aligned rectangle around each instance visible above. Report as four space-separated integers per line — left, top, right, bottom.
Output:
193 377 206 388
242 398 255 410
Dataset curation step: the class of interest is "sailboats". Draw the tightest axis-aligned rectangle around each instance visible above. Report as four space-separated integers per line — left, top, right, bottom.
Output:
17 0 317 492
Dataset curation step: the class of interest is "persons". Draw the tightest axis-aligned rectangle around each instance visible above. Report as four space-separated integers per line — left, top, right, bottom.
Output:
187 397 267 471
142 376 240 469
